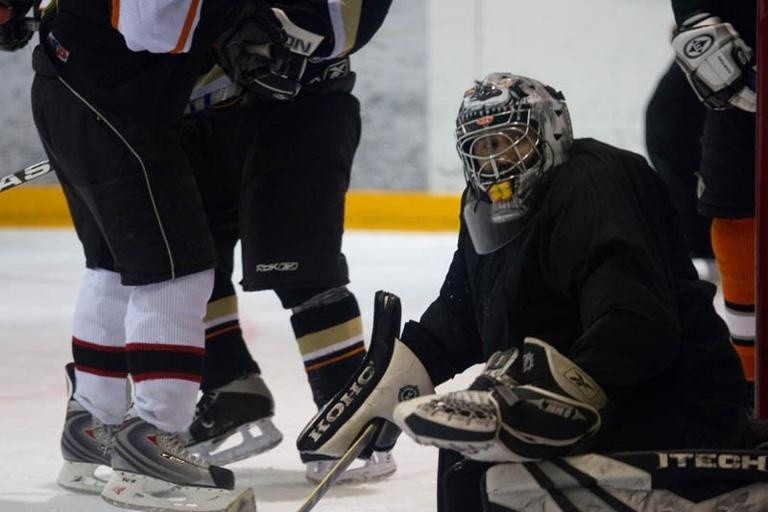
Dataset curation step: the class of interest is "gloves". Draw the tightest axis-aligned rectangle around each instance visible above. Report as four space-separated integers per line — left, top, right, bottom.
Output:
670 11 757 115
0 0 42 52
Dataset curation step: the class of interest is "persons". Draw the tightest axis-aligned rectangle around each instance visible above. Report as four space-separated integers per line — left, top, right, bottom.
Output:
645 0 762 394
398 73 756 511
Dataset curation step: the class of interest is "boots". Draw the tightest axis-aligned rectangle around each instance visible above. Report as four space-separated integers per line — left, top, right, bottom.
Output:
61 361 276 492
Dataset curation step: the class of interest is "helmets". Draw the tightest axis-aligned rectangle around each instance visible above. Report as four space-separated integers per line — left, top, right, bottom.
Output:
455 71 575 203
